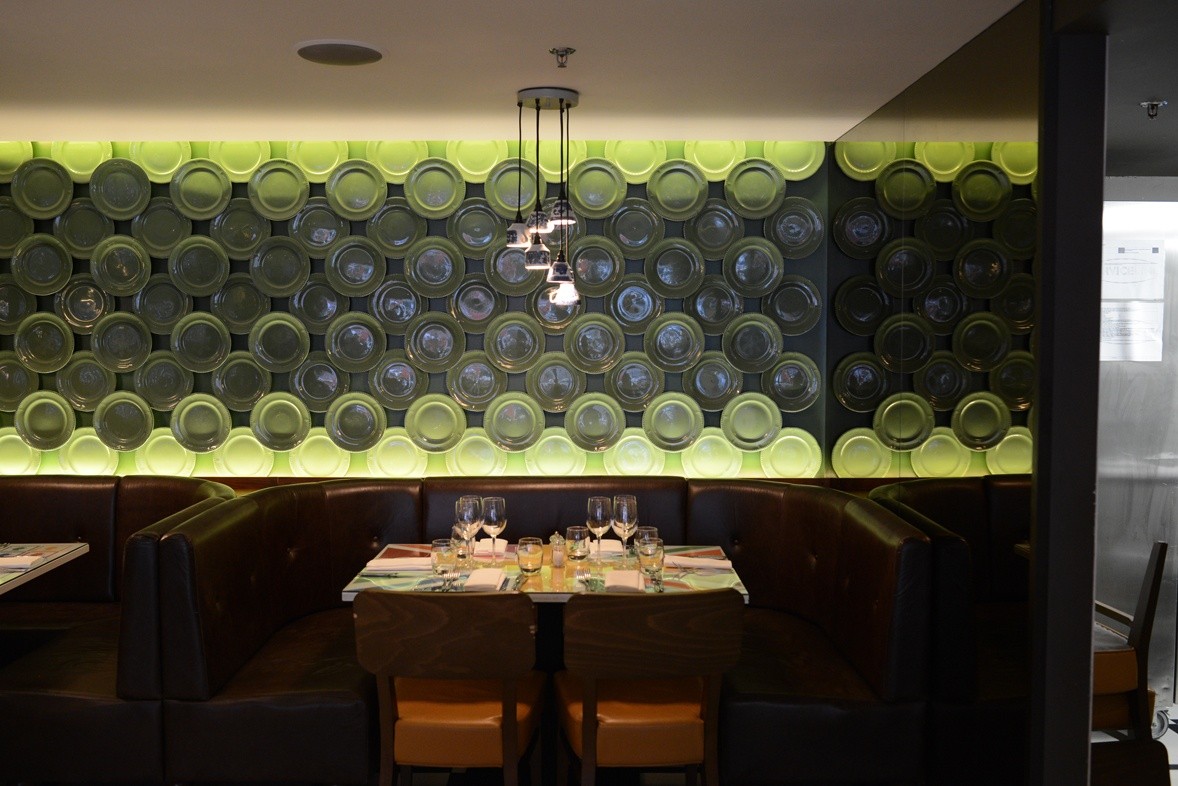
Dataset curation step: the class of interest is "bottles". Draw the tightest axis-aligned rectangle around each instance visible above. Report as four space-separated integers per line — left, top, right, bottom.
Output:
549 530 568 568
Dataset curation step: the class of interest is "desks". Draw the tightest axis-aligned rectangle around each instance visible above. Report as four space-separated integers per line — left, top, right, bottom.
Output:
342 543 749 672
0 542 89 596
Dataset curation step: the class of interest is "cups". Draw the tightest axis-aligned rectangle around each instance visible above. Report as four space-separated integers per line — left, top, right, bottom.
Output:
432 539 453 575
518 537 543 575
633 526 659 557
639 537 664 574
565 525 591 559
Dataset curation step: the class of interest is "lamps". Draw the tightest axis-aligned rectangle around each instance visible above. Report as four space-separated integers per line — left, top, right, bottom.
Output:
505 86 580 305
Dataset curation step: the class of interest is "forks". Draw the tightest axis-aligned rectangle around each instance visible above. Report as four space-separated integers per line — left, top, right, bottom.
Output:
576 568 592 591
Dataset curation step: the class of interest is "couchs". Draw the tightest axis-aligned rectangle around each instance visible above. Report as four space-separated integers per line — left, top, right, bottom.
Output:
869 479 1028 786
0 474 936 786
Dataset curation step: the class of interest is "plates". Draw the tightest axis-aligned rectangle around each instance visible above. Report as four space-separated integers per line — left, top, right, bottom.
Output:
833 140 1037 478
1 143 825 477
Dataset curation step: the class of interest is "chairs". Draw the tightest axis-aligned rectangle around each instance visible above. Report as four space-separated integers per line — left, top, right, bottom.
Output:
554 587 744 786
1090 541 1172 786
352 587 549 786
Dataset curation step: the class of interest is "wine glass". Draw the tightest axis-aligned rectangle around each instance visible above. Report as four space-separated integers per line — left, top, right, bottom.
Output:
586 497 612 558
455 494 481 569
481 497 508 567
611 494 638 569
